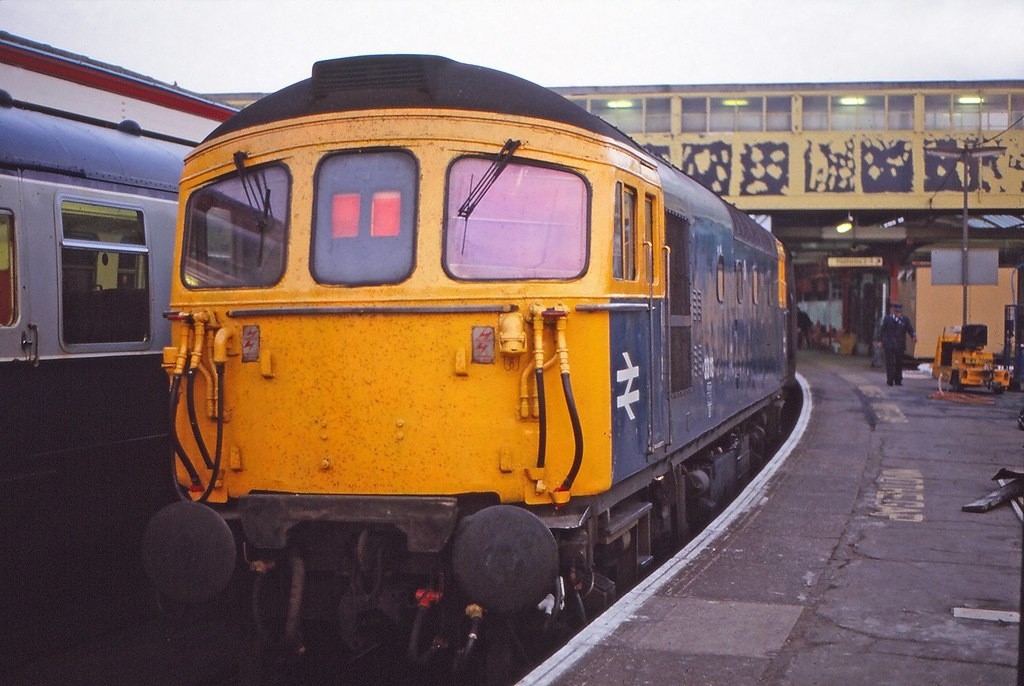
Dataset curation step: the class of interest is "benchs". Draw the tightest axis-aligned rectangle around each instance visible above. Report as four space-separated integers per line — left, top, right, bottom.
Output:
63 288 149 344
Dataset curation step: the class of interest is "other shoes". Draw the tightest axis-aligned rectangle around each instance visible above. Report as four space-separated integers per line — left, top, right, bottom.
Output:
896 381 902 385
887 380 893 385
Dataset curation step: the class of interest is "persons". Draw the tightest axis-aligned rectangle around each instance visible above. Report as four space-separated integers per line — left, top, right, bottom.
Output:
796 306 813 350
877 302 918 386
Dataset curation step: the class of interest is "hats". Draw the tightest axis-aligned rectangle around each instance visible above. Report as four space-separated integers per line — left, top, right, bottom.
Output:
894 303 904 312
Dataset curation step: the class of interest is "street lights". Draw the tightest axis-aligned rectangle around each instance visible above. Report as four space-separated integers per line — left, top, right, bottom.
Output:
924 145 1007 335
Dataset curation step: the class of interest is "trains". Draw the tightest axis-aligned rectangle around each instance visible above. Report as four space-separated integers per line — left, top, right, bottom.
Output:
0 31 288 535
142 55 799 672
